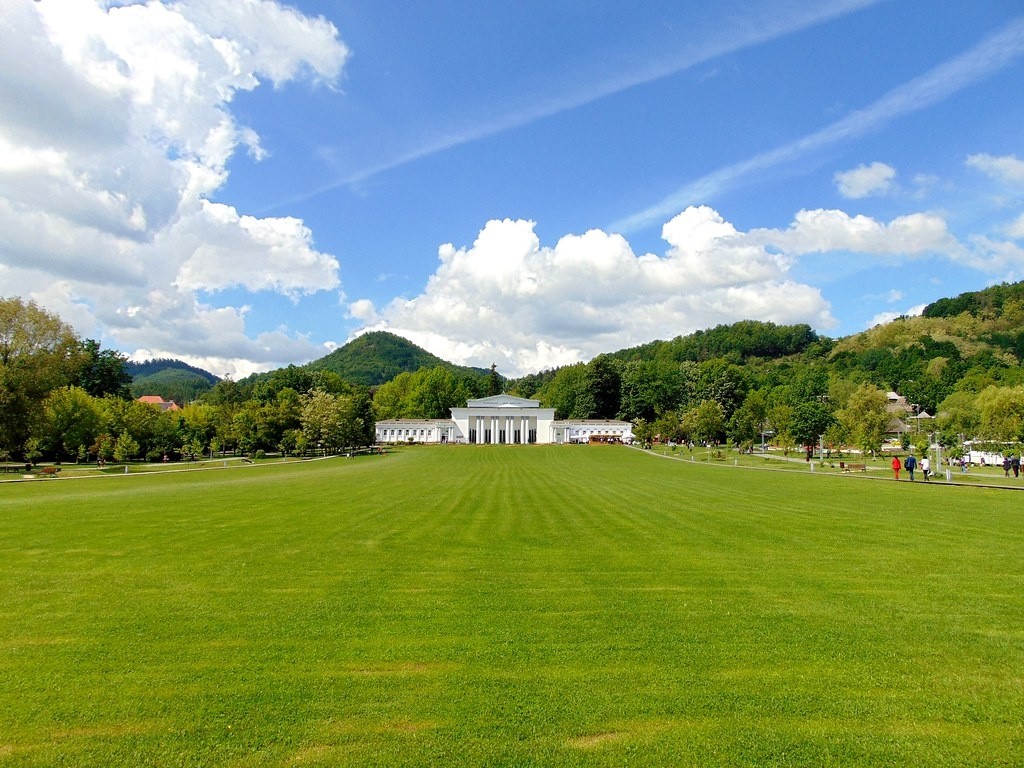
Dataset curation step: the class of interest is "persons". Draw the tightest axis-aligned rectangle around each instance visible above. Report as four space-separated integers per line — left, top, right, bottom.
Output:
1003 455 1020 477
959 459 965 473
734 442 754 455
685 439 694 452
376 447 384 457
440 438 461 445
600 436 636 446
699 438 721 448
346 451 355 462
892 454 930 481
360 446 374 455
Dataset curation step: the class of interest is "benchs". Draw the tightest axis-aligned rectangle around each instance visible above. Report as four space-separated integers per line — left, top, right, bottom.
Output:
37 467 57 478
844 464 867 473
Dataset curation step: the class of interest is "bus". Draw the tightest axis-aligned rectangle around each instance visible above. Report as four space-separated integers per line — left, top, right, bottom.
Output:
962 440 1024 467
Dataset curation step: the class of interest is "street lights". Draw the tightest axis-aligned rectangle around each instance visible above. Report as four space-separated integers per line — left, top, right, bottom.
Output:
912 404 921 433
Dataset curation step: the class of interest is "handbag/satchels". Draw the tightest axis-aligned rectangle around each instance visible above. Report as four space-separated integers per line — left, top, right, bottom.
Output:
908 457 910 467
927 470 935 477
